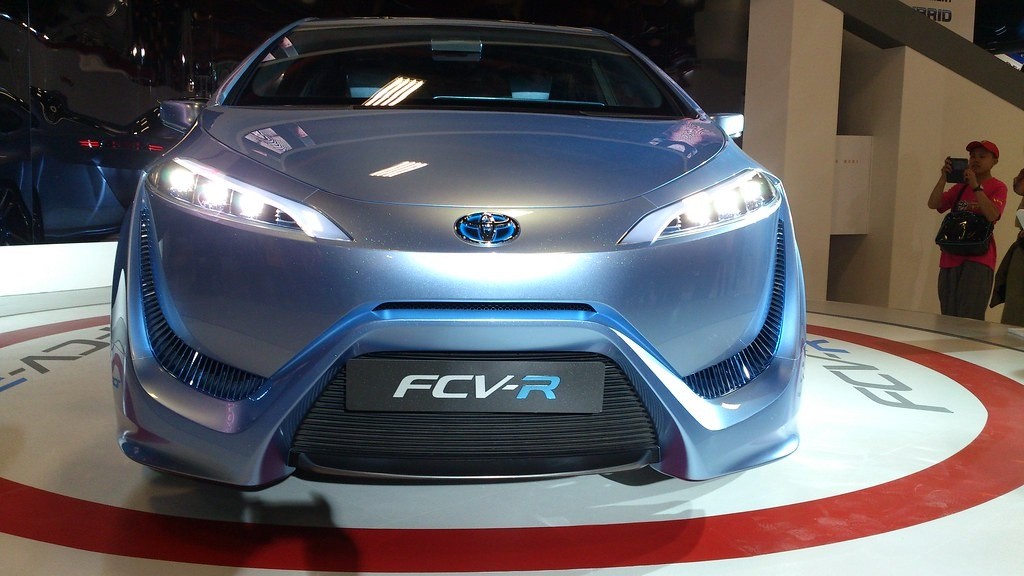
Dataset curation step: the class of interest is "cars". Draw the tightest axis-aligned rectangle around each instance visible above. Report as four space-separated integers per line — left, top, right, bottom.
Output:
0 82 211 246
109 15 809 494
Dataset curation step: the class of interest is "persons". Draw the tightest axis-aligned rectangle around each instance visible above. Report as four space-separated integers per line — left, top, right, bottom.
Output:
928 140 1008 321
990 168 1024 327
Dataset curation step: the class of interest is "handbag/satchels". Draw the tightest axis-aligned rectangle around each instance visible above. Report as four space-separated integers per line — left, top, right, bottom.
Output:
935 211 994 257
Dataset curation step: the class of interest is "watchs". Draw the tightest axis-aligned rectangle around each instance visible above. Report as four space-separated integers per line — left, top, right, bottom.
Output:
973 184 984 193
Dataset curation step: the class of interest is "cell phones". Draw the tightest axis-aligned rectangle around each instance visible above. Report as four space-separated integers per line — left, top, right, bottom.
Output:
946 159 968 183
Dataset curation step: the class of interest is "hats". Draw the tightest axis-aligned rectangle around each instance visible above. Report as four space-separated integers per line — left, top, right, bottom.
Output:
966 140 999 159
